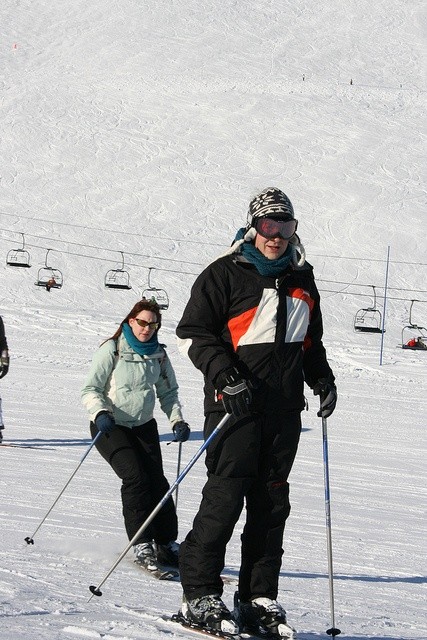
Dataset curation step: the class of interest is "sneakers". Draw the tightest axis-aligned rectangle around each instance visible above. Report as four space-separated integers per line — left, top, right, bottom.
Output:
181 592 239 635
158 541 182 564
234 591 286 626
133 542 159 571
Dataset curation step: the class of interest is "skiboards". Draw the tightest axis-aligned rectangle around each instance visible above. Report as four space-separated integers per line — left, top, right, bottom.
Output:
134 541 238 584
115 604 307 640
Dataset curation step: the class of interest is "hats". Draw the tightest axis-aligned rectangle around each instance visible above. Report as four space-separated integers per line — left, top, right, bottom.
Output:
243 187 301 248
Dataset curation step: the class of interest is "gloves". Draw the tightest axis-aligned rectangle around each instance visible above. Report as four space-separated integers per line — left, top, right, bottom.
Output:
214 367 254 417
313 379 337 418
95 412 116 435
175 422 190 442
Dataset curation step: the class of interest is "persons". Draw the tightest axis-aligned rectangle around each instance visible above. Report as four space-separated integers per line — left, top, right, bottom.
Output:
0 316 10 442
78 300 190 571
176 188 337 640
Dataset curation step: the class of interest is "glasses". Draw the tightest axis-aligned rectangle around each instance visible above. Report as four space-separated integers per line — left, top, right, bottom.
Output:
134 316 159 329
246 212 298 241
